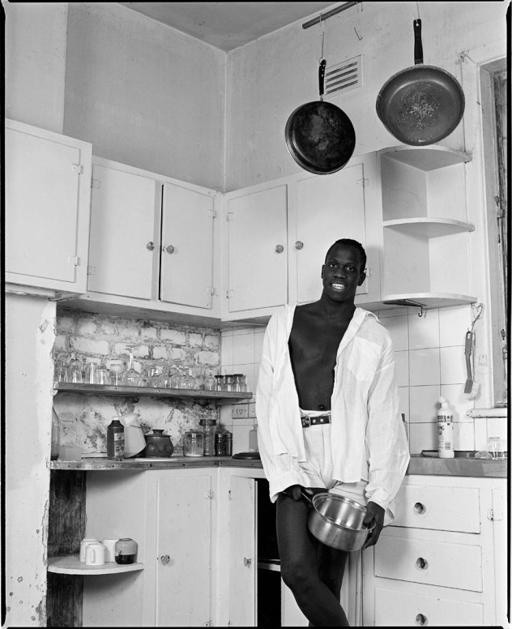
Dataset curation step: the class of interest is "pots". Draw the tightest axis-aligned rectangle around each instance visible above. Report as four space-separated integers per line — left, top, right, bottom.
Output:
143 429 174 457
375 17 466 146
300 487 377 552
284 59 356 175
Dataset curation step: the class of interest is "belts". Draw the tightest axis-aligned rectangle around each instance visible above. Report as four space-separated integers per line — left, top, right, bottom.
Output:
301 416 329 428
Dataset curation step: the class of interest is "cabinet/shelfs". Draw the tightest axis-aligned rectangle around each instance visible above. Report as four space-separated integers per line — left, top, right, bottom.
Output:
376 143 481 311
2 117 93 302
82 467 507 627
214 150 408 330
54 155 222 328
82 467 507 629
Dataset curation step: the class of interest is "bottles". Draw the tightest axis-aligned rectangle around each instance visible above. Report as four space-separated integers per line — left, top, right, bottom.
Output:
215 374 245 391
437 396 455 458
51 388 60 461
183 418 232 457
107 415 125 460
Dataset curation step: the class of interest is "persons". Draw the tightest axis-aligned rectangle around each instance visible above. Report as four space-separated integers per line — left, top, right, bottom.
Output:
253 236 411 627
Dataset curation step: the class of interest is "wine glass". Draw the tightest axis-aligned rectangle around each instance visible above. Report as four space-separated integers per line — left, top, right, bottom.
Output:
55 344 215 392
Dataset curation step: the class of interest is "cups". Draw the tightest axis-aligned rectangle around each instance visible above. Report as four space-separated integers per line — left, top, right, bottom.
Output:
79 538 138 566
